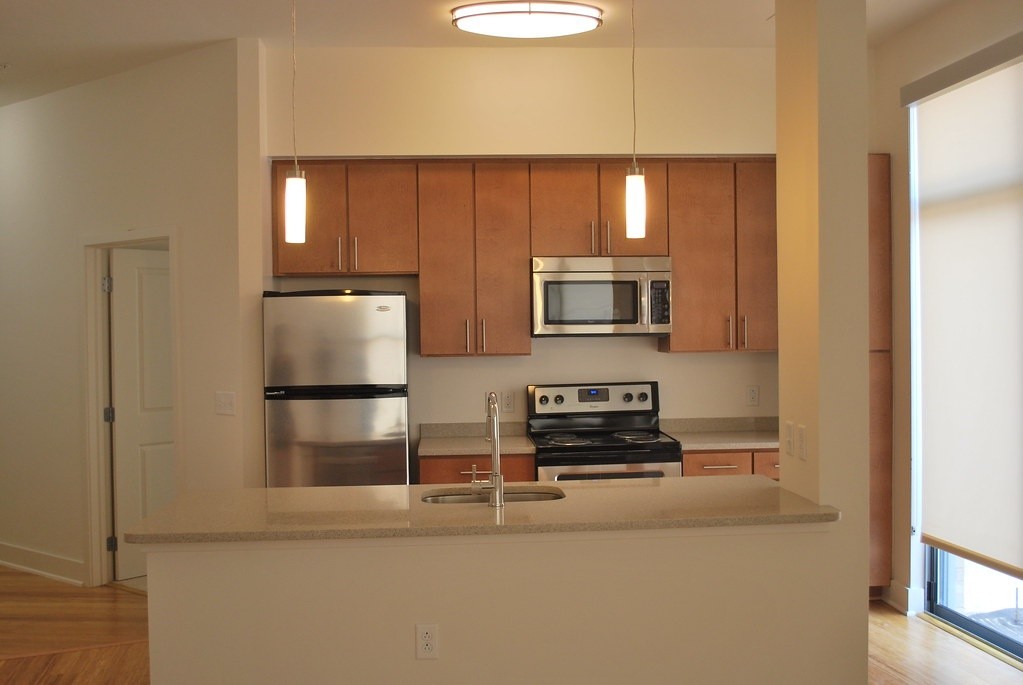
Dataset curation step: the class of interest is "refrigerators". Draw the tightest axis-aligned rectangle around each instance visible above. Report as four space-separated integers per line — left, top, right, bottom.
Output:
263 291 411 485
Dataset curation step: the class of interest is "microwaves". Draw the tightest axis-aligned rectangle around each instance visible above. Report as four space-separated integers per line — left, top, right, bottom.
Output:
531 257 673 334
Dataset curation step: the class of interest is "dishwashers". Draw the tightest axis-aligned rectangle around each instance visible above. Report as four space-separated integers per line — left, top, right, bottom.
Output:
536 453 683 481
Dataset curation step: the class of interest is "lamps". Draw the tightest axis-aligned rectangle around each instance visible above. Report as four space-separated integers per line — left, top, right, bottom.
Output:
625 0 647 239
283 0 306 243
450 0 604 38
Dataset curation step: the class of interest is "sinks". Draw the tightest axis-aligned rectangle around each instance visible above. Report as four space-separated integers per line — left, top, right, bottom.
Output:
421 485 566 504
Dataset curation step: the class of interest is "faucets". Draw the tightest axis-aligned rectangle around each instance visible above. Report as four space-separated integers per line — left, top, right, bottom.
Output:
481 391 505 507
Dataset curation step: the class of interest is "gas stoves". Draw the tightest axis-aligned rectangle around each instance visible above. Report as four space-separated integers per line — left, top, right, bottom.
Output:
528 430 682 455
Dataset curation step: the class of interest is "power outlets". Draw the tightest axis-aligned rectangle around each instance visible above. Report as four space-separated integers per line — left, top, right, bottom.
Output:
501 392 514 412
414 623 439 660
746 386 759 406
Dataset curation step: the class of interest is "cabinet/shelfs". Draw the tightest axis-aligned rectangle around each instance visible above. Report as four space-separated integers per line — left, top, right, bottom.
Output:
682 451 780 481
418 456 536 484
870 352 893 587
418 162 532 355
272 163 419 276
530 162 668 257
656 161 779 354
869 153 892 352
